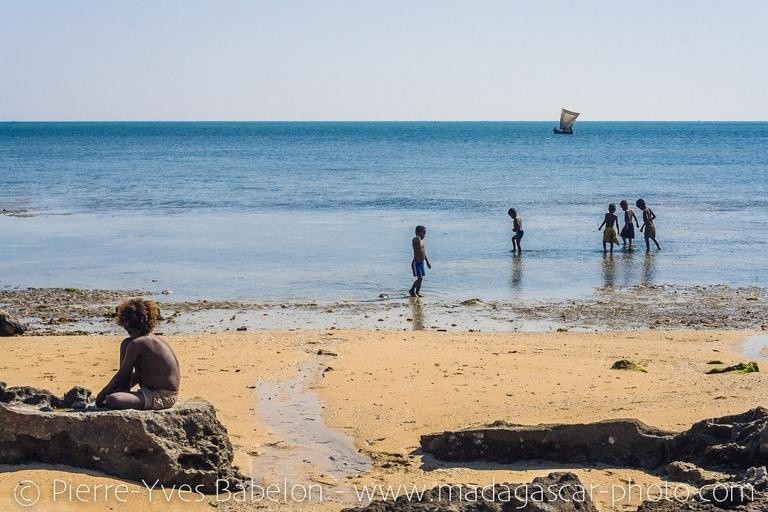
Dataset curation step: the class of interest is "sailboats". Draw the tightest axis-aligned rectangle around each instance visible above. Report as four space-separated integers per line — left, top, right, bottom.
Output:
553 109 580 134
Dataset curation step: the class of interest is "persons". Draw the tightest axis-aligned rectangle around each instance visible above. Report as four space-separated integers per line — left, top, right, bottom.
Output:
618 199 640 246
598 202 620 255
635 199 662 253
408 225 432 298
94 297 182 410
507 207 524 255
